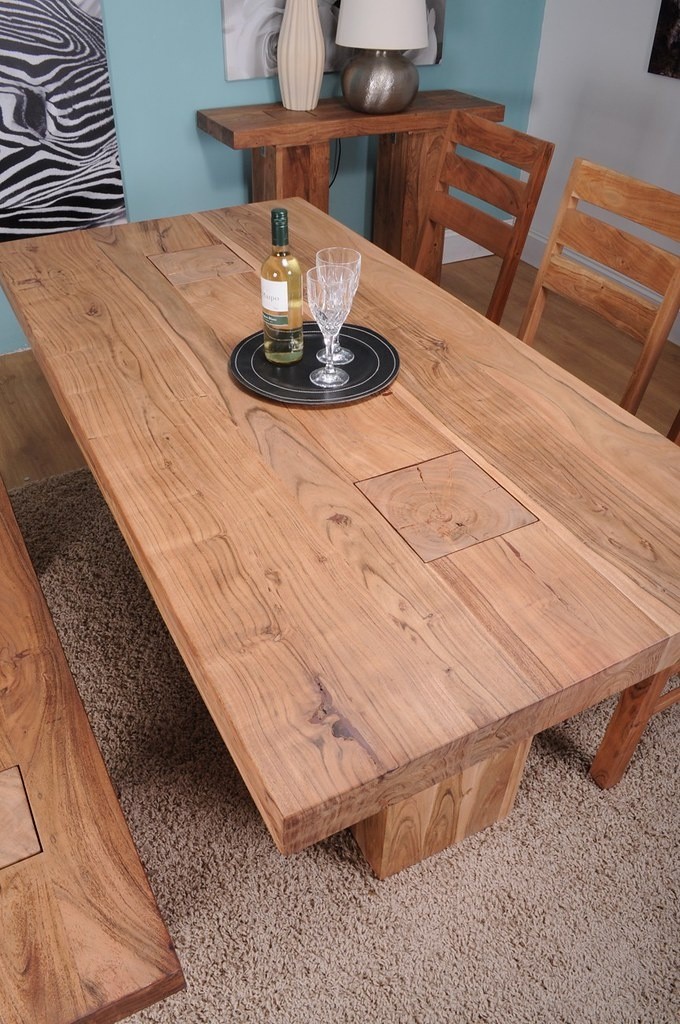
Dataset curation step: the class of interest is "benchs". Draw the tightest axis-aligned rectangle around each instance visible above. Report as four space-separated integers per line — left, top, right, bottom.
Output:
0 476 187 1024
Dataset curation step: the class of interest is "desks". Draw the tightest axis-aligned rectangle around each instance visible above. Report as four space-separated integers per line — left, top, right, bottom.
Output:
0 196 680 880
196 89 508 262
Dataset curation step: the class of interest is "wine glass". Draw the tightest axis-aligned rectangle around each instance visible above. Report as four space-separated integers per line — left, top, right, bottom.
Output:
314 246 363 364
306 265 355 389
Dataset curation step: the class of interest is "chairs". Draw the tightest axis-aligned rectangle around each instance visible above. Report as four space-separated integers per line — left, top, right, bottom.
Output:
409 109 556 326
520 157 680 417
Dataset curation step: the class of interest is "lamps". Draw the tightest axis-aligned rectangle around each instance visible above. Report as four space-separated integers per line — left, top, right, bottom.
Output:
334 0 429 113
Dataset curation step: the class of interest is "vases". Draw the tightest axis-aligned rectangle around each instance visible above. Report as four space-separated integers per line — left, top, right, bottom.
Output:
277 0 323 111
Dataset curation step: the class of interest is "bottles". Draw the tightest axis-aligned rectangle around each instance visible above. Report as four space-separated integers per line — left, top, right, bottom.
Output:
259 208 308 364
